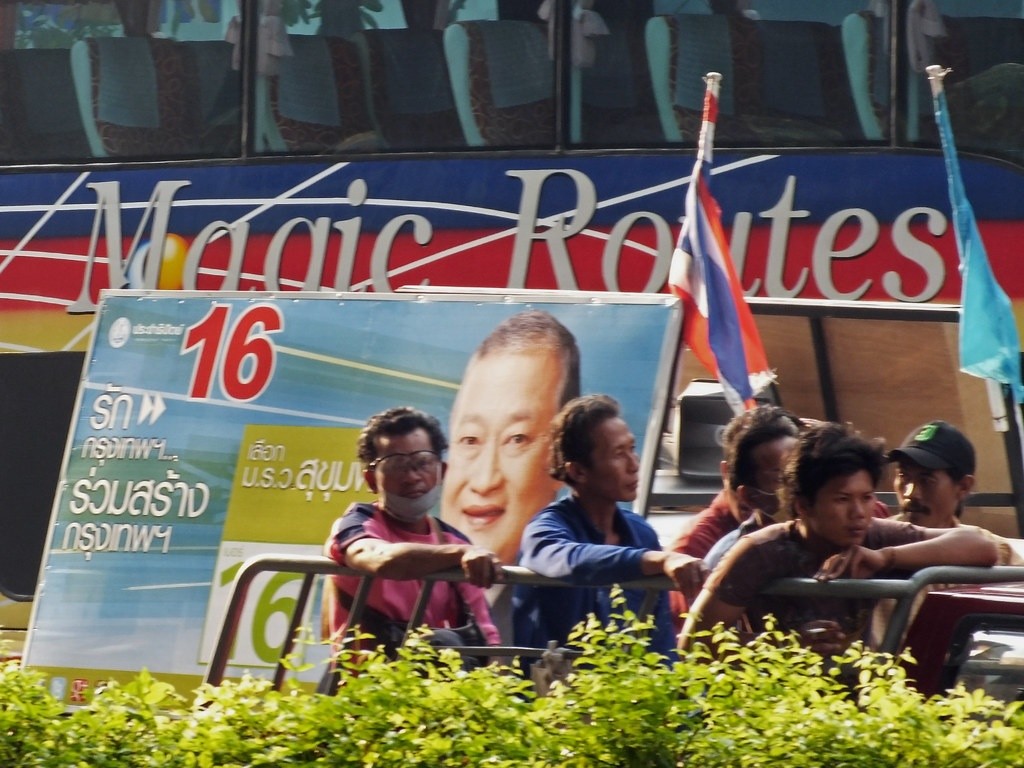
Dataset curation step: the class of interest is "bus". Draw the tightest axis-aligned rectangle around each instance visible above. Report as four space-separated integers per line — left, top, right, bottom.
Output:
0 0 1024 629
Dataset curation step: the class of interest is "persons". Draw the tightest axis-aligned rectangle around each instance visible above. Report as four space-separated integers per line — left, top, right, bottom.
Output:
701 417 805 572
324 406 506 676
438 308 582 677
871 421 1024 662
661 405 891 649
511 393 713 698
679 422 1014 708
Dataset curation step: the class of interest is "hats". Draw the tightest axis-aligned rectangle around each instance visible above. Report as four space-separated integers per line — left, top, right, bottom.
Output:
886 419 976 475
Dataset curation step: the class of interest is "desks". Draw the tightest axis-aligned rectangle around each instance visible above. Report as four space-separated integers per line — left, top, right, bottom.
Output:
649 470 720 549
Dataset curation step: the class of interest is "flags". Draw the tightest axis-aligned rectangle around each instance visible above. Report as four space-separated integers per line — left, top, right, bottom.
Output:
666 92 780 418
933 87 1024 411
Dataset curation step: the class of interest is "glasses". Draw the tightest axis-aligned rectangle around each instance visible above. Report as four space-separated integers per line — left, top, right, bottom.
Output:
369 449 442 479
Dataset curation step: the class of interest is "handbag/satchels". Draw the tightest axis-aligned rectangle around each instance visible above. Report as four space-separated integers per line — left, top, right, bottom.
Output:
382 616 489 674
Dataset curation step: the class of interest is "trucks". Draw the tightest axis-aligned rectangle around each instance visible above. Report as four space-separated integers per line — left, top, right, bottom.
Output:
188 555 1024 733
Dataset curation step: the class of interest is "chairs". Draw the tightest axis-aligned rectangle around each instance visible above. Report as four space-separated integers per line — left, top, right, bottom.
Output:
271 33 373 150
443 20 554 146
754 19 868 139
185 40 244 155
357 28 467 149
70 36 204 155
1 49 91 157
906 0 986 82
647 13 843 144
583 24 665 144
841 10 1023 153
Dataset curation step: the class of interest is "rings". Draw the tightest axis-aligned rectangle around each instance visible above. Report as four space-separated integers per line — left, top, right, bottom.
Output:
841 555 848 560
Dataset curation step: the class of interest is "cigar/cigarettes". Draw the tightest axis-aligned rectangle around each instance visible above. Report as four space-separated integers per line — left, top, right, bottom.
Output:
806 626 838 634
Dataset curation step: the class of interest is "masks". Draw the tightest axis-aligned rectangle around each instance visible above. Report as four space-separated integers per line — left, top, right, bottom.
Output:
378 463 441 523
748 489 794 523
